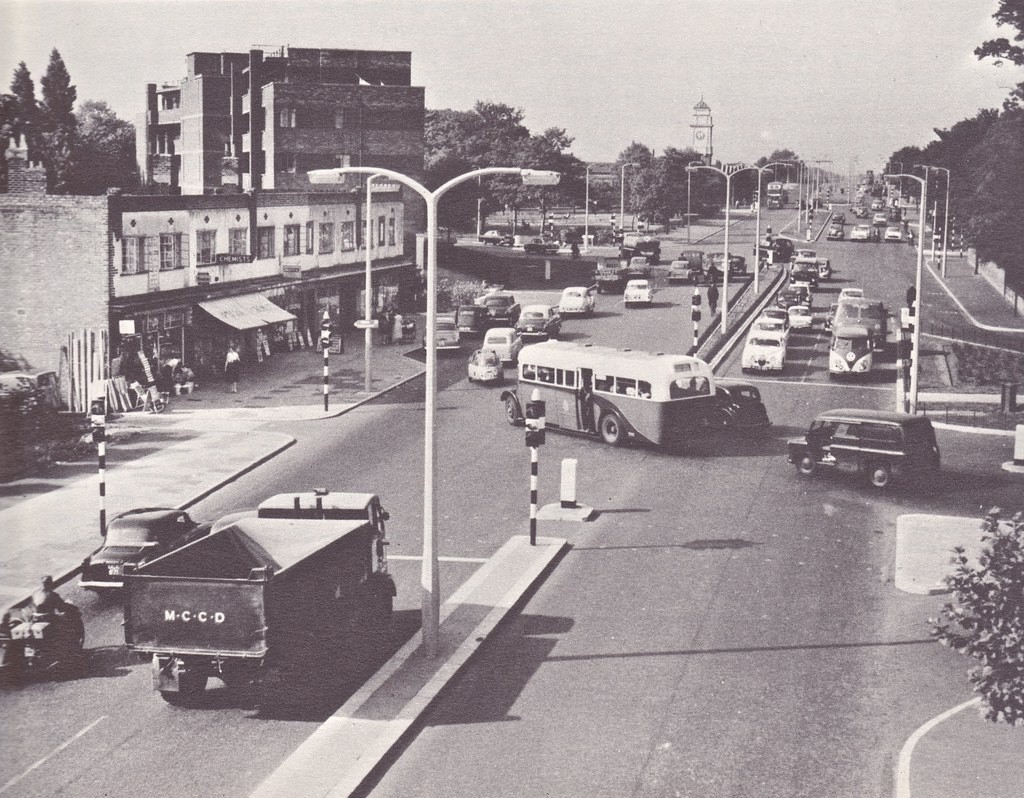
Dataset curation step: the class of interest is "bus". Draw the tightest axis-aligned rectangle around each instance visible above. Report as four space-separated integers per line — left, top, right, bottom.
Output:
765 181 784 209
498 337 717 451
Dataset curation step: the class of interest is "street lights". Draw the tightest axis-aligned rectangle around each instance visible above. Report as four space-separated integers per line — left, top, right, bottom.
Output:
880 171 929 415
744 152 836 296
618 161 641 256
684 164 774 334
685 159 704 244
894 162 950 278
582 164 599 251
308 166 560 662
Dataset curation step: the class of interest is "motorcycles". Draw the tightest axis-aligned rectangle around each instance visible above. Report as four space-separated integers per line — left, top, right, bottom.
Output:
0 601 86 680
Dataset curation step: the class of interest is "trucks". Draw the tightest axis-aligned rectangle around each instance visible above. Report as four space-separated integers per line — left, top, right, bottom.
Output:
118 487 398 710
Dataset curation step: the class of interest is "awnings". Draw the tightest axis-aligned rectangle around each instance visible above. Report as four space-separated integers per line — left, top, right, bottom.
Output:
199 293 297 331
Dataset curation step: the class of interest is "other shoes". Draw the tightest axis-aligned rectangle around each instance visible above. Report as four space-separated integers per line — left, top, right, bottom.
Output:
231 388 237 392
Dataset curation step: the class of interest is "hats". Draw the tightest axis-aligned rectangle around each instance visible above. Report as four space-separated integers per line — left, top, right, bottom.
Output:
40 576 54 583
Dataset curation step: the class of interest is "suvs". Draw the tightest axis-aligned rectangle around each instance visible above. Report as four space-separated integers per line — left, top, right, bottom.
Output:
785 405 940 494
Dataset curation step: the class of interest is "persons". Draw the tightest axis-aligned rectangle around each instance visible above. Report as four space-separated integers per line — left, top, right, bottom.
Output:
874 197 914 244
33 576 71 615
380 309 403 344
570 239 579 259
169 347 241 395
678 253 719 313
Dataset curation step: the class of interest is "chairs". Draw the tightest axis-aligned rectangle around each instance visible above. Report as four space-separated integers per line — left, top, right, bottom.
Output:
130 381 164 414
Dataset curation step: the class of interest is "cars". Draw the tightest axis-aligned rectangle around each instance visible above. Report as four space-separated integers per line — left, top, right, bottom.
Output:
556 286 595 317
523 234 559 257
716 381 771 437
885 226 903 243
478 230 515 246
850 224 871 242
77 507 212 605
420 285 596 382
826 224 844 241
596 235 885 382
831 169 902 226
